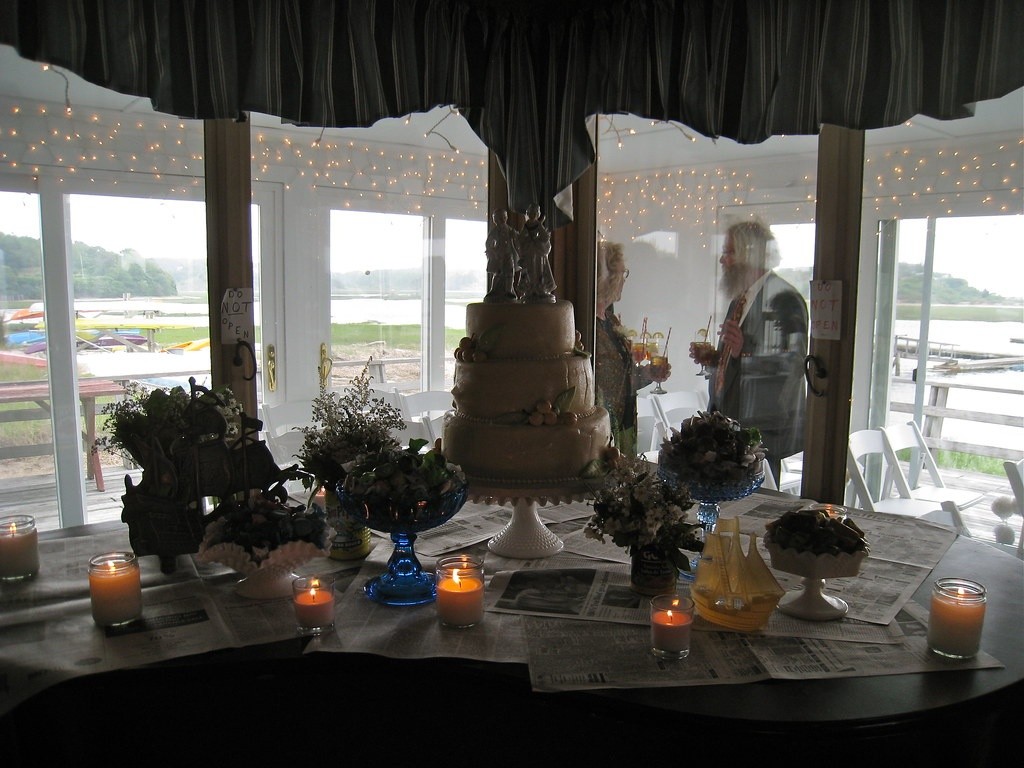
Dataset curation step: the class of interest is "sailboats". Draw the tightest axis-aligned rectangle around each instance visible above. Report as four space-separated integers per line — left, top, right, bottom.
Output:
689 514 787 633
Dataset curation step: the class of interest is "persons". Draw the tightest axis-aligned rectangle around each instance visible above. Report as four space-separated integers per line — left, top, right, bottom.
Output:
690 221 809 492
591 240 671 459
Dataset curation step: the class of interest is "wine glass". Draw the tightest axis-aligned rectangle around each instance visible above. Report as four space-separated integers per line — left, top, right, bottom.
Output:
646 337 659 365
650 346 668 394
695 332 711 376
632 338 647 365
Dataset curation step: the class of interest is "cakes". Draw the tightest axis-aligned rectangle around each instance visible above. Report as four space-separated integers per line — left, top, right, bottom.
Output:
441 202 613 482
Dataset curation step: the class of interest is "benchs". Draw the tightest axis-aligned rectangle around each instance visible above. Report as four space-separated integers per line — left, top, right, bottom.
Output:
0 378 128 492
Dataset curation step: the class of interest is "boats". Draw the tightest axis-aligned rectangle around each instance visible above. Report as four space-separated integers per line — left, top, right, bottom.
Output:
2 301 211 358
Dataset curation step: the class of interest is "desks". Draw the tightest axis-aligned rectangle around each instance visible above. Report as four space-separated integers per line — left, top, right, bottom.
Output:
0 447 1024 768
32 319 197 355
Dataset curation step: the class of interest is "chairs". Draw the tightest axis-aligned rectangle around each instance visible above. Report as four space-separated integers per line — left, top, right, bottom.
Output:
1000 451 1024 549
645 388 802 499
259 388 454 470
846 421 987 540
